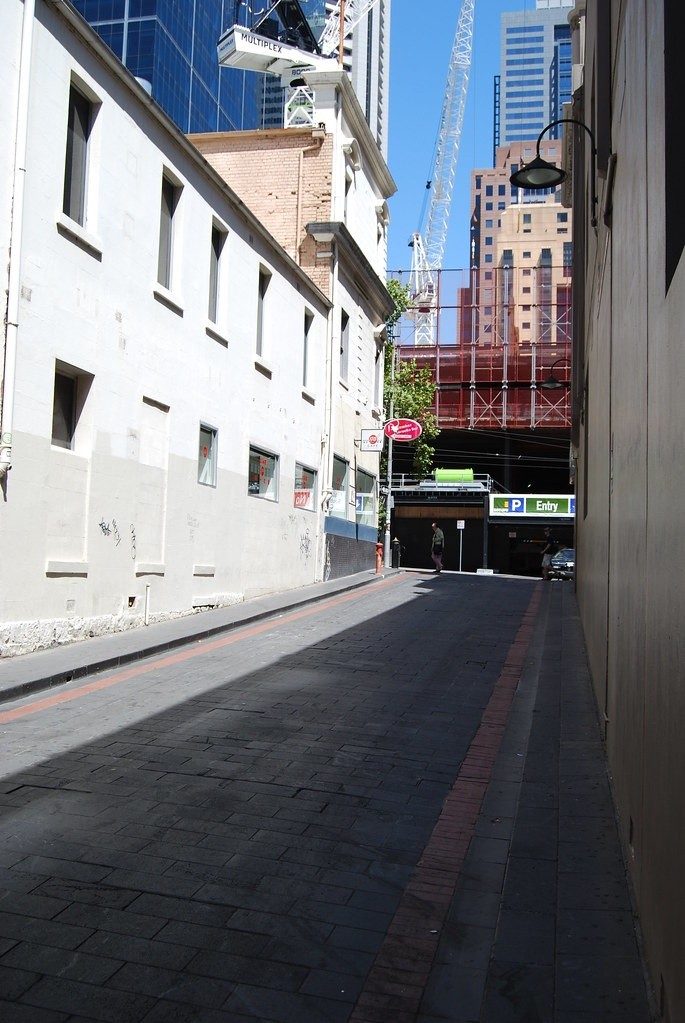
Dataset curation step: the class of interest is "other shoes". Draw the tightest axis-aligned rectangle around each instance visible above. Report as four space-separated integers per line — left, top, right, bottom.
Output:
433 565 443 573
542 579 549 580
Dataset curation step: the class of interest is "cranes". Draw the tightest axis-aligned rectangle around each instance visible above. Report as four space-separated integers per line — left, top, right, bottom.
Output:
405 0 479 348
216 0 382 128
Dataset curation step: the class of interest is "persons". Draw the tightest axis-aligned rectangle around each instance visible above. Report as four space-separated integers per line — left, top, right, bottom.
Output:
540 527 560 581
430 523 444 573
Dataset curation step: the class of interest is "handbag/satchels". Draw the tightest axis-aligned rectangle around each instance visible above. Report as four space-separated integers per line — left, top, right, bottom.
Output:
433 544 442 555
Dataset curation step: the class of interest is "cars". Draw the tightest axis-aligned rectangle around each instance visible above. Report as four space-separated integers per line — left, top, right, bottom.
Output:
543 548 576 580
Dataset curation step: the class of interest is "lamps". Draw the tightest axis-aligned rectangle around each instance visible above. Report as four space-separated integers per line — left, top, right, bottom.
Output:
541 359 571 389
509 118 597 227
